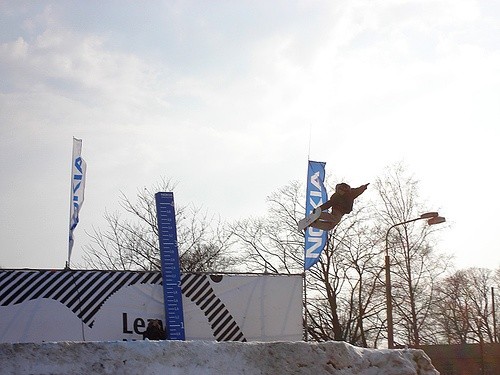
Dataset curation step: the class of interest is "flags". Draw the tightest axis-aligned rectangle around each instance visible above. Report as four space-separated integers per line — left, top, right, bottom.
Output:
59 129 93 261
296 152 338 294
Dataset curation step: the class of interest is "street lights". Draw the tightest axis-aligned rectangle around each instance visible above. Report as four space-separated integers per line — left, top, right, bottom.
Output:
385 212 446 350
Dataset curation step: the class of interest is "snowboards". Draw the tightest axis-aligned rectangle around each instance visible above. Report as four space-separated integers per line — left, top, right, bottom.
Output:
298 207 322 232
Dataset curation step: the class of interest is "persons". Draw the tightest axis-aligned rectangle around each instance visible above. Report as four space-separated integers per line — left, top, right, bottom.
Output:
309 183 370 231
